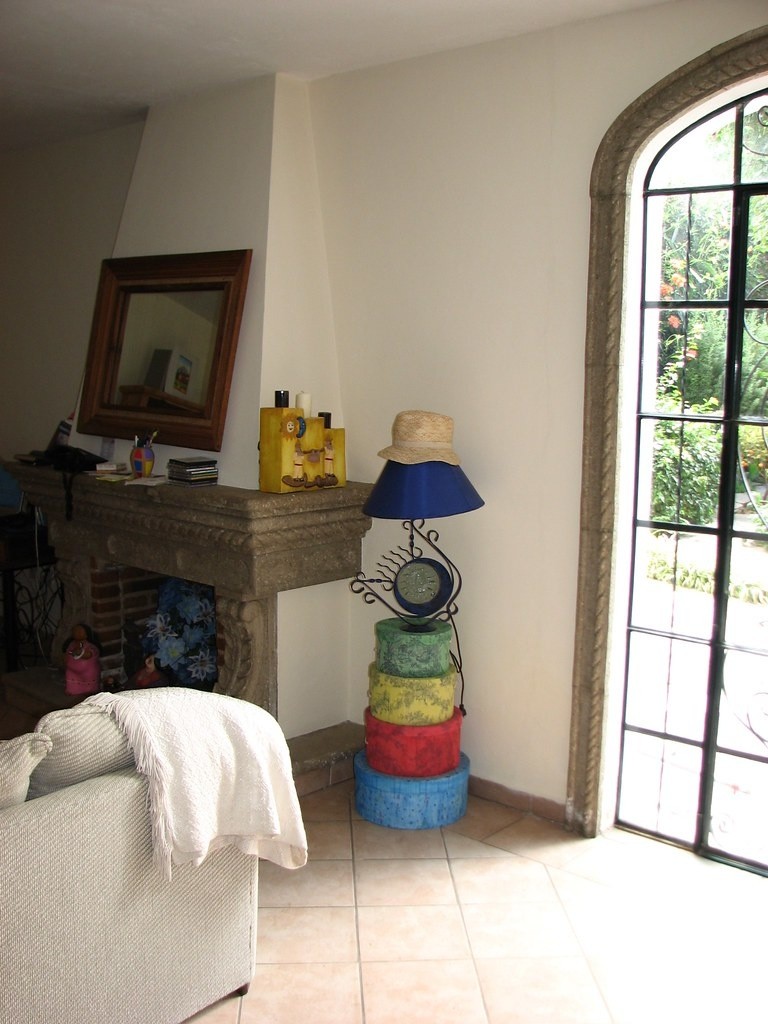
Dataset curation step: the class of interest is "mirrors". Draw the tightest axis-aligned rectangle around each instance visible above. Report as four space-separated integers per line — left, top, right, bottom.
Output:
76 249 253 453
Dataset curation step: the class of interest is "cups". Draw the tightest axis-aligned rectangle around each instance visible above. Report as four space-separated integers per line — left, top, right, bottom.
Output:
100 438 115 462
275 391 289 407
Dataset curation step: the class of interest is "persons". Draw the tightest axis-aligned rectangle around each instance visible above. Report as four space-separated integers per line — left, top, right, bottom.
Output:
65 624 102 694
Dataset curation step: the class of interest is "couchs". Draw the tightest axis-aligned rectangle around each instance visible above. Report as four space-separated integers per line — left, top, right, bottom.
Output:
0 688 311 1024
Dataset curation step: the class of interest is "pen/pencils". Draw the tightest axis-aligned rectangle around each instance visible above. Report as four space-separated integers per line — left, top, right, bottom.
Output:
132 426 159 450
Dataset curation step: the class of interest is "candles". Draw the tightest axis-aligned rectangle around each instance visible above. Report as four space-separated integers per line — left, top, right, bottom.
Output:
297 391 312 418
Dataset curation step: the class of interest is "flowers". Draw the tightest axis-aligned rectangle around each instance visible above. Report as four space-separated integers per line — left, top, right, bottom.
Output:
141 577 217 687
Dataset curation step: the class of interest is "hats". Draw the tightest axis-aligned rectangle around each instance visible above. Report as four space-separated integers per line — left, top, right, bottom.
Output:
378 410 461 465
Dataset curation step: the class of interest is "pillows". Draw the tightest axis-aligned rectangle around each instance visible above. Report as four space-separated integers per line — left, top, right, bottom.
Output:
26 696 212 802
0 731 53 810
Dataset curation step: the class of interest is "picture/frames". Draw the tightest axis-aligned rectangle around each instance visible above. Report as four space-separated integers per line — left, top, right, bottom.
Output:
164 348 198 400
